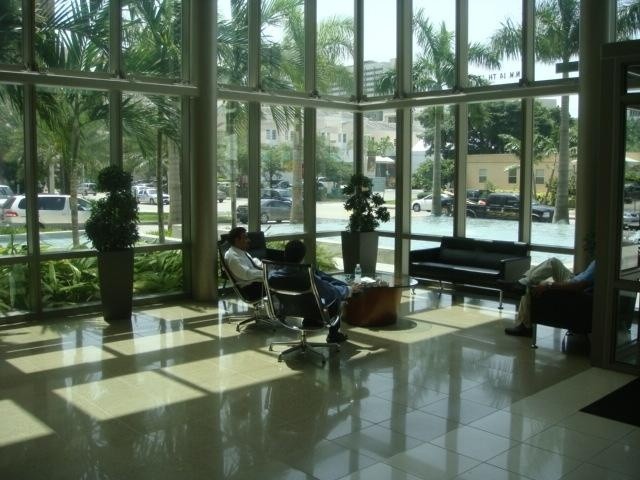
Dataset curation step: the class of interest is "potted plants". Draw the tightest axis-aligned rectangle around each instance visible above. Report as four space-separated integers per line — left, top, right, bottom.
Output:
341 174 390 276
83 164 141 323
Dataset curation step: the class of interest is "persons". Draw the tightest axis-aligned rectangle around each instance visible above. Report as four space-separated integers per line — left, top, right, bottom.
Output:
268 239 366 344
504 255 597 338
225 227 274 302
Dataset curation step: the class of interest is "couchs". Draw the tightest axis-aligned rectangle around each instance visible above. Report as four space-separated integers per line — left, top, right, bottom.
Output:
220 231 285 298
409 235 532 310
525 278 595 349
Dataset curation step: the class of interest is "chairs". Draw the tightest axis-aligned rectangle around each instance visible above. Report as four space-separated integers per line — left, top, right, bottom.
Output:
261 259 343 366
217 240 285 332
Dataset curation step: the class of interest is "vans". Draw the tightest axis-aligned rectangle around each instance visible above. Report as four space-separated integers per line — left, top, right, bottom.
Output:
2 194 99 225
218 181 239 187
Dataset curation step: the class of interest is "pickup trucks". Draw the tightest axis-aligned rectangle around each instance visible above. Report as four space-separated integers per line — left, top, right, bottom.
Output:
447 192 553 222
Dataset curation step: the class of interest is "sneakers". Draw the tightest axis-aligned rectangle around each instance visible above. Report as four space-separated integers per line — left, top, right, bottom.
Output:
504 322 533 338
497 278 527 295
326 332 347 343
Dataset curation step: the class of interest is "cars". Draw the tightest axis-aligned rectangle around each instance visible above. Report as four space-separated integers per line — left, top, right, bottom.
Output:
136 188 169 204
623 210 640 229
263 188 293 201
317 177 347 193
77 182 99 194
410 193 449 212
466 189 492 198
236 199 291 223
0 185 13 212
217 190 227 202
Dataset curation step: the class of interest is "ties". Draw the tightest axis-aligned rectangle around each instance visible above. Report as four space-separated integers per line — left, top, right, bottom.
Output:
245 252 263 270
